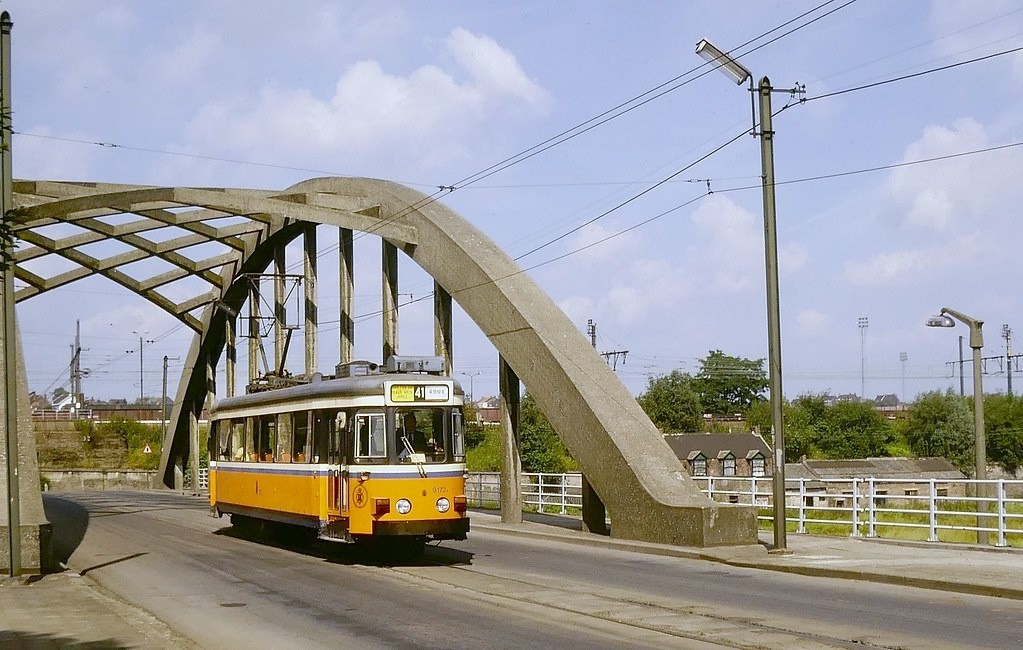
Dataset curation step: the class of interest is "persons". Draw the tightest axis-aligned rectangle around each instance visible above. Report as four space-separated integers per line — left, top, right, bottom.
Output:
396 413 429 457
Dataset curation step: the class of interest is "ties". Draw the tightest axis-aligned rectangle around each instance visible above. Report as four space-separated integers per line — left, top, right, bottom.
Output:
408 433 412 445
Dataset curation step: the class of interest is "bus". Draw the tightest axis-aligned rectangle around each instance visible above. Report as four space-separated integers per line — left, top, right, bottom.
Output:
208 356 470 557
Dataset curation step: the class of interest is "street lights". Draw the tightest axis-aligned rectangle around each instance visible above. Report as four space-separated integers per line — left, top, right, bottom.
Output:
858 317 868 397
162 355 180 444
695 37 788 550
900 352 908 400
925 308 990 546
462 372 481 409
